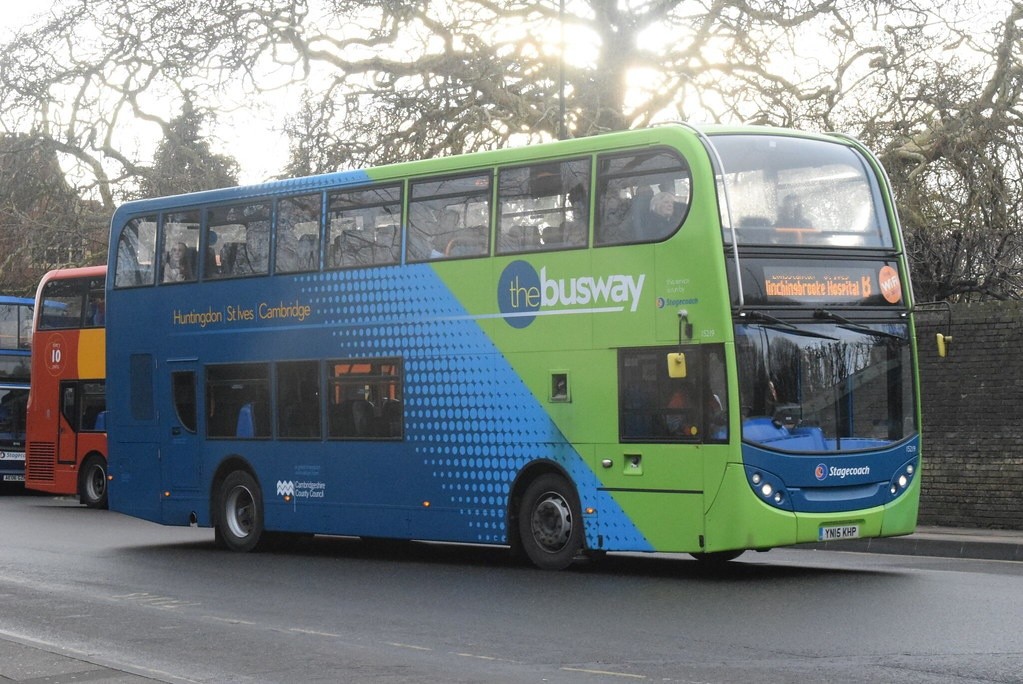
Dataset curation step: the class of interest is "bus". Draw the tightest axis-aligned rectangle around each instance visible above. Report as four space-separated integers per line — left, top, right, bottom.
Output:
0 294 77 497
23 263 398 512
101 117 956 573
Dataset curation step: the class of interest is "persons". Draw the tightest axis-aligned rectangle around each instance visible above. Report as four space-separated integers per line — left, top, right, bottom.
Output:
150 163 691 281
769 194 820 245
236 378 337 440
665 370 745 435
93 301 105 325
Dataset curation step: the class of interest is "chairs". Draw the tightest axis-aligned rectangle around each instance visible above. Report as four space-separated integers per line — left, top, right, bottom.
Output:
164 224 573 281
177 397 402 438
